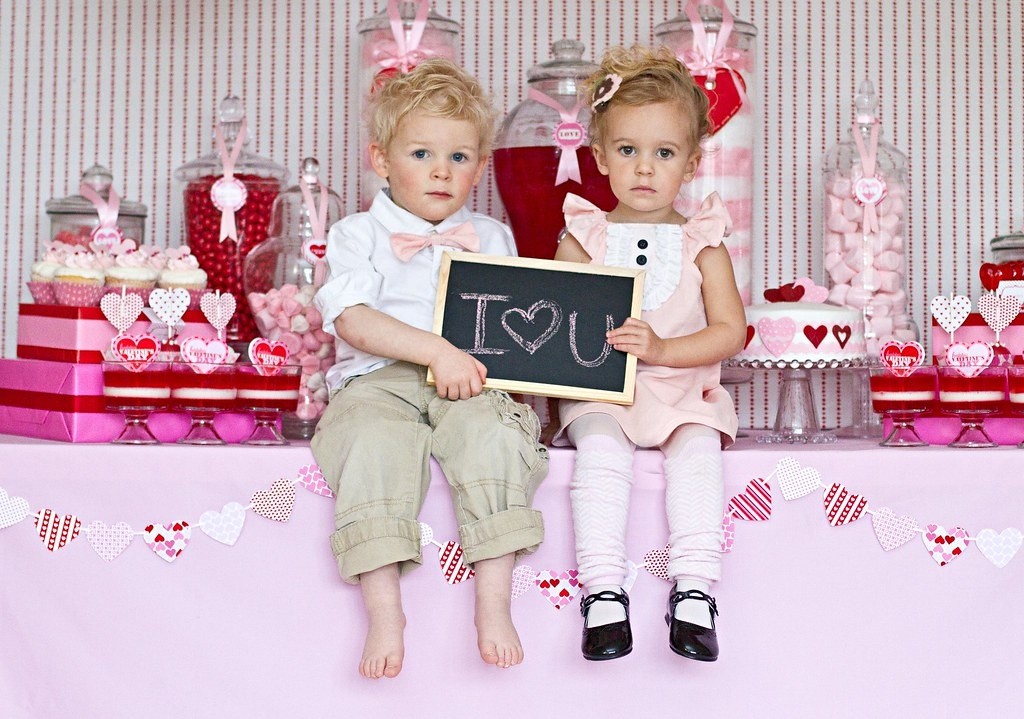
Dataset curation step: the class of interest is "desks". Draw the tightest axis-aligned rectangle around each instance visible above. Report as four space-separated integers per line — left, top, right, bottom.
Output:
1 430 1024 719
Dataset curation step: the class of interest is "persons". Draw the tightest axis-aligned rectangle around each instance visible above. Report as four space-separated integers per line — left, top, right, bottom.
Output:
540 45 748 661
310 58 549 679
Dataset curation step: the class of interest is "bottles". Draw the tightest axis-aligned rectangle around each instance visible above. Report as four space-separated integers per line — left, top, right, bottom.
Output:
243 157 347 441
174 94 293 357
492 39 618 258
654 0 759 439
359 0 463 212
989 229 1024 264
821 79 915 441
45 163 150 250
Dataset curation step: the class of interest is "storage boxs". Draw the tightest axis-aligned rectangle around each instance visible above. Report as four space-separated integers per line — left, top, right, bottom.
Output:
17 303 227 366
0 358 284 446
926 311 1024 369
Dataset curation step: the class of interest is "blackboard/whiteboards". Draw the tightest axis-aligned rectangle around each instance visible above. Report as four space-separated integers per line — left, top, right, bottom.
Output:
424 246 648 408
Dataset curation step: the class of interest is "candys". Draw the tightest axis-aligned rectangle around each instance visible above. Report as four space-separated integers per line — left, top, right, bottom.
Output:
248 282 337 423
181 175 286 341
819 171 918 360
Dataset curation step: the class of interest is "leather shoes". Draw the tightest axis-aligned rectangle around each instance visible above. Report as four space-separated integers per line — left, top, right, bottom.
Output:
580 586 633 662
664 583 719 661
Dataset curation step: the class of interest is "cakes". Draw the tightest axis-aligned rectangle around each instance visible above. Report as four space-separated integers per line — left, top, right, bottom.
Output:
728 277 865 364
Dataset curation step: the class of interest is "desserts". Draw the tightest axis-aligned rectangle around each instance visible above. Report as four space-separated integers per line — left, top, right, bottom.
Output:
20 241 213 310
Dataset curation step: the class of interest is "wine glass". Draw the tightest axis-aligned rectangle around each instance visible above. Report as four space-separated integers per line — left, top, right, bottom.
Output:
1008 364 1024 447
937 364 1010 448
101 361 301 446
868 364 939 446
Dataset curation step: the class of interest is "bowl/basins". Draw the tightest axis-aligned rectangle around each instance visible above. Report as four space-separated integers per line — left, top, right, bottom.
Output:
27 280 214 311
